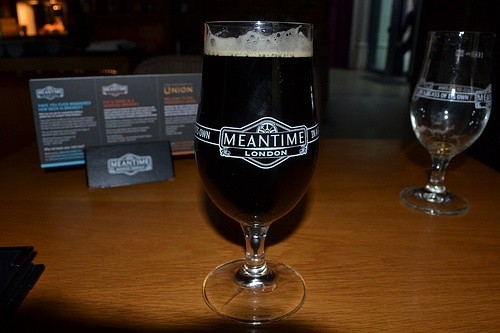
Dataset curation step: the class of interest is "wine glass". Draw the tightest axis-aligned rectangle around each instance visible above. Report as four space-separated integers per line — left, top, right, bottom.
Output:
400 31 491 215
195 20 319 326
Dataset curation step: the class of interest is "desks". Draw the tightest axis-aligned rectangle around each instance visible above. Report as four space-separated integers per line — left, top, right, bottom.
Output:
1 133 500 333
